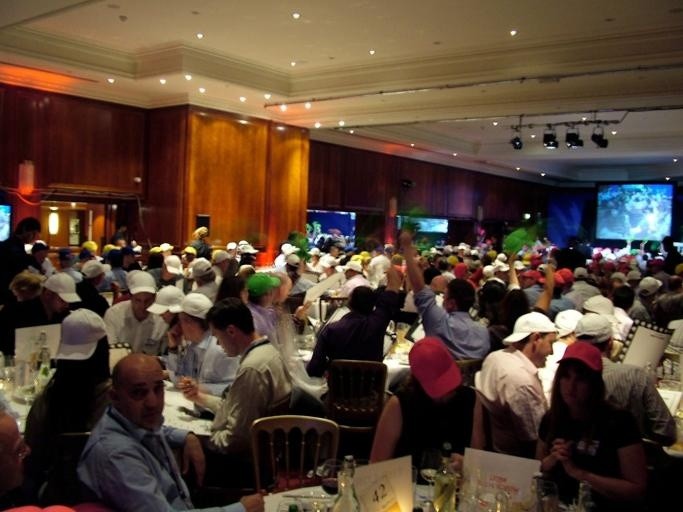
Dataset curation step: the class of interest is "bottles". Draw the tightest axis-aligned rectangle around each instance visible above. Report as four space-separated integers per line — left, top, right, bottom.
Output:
37 330 52 393
473 473 591 512
330 454 364 511
433 443 454 511
409 466 434 511
646 360 680 386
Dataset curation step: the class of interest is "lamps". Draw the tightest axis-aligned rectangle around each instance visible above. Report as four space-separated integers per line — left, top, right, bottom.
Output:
506 114 629 154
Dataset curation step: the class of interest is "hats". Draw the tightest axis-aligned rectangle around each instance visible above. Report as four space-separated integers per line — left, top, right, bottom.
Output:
24 240 683 399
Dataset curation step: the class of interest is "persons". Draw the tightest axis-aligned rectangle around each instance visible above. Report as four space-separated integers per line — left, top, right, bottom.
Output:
471 313 557 458
574 313 679 446
72 350 266 512
0 392 33 512
177 298 292 485
24 306 112 505
2 217 683 439
535 342 650 512
367 334 489 484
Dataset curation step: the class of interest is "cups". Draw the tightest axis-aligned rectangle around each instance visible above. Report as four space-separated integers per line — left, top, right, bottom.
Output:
278 490 327 511
659 380 681 406
663 408 683 457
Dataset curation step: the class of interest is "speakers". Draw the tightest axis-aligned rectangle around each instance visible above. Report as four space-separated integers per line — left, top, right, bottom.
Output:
195 215 210 230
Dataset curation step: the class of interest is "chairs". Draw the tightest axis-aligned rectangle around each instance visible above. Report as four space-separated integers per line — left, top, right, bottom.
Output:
0 294 681 512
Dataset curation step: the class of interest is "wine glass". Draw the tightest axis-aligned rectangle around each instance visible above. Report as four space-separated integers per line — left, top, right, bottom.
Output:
0 353 35 418
